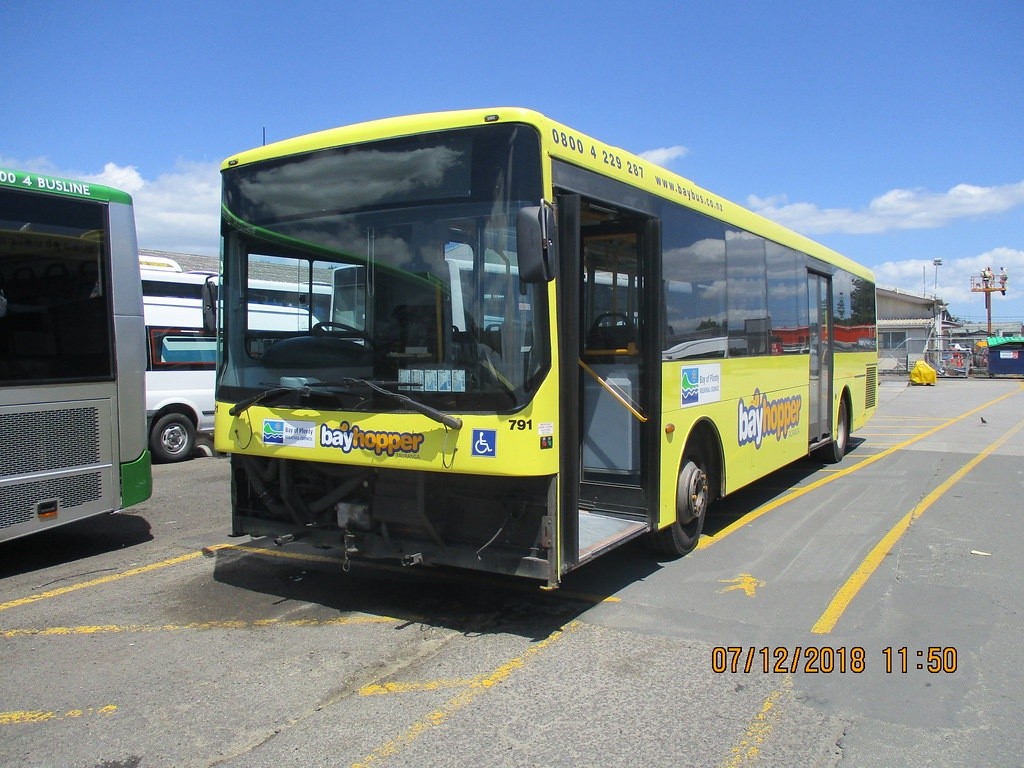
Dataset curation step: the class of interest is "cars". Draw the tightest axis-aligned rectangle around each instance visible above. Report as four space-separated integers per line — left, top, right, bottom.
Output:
975 347 989 367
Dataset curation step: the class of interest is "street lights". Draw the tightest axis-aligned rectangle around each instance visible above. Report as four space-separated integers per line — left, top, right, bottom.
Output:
938 302 949 360
933 257 942 299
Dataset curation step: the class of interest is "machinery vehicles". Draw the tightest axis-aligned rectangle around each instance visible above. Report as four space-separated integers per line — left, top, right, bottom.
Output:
970 272 1007 354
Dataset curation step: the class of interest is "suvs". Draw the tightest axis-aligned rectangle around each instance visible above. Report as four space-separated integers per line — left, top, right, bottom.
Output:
947 343 971 352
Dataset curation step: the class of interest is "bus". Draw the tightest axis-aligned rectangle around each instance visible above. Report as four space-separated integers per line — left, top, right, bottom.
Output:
336 264 718 373
200 107 876 605
140 253 331 467
0 168 155 539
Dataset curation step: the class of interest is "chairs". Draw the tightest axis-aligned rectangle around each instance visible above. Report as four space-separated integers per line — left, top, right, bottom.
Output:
452 321 533 355
586 313 636 361
